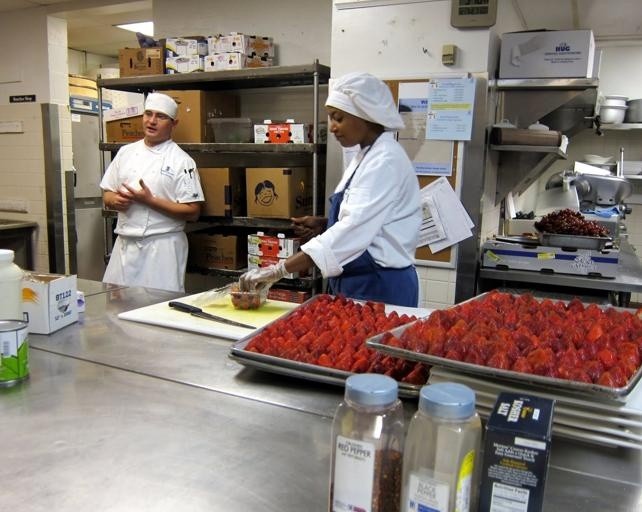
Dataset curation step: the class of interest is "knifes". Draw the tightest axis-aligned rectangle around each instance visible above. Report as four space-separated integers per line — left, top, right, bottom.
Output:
168 298 260 330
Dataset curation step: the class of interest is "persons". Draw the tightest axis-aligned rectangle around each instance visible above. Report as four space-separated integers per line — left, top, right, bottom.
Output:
99 92 206 292
239 71 421 306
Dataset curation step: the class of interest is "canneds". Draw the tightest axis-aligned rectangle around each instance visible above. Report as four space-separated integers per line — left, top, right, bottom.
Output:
0 319 29 385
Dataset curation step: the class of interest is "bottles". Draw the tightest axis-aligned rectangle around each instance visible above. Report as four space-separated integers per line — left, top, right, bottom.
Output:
329 372 482 512
0 250 23 322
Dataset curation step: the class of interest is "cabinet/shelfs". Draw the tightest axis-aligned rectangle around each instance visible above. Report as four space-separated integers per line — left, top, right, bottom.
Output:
95 60 331 304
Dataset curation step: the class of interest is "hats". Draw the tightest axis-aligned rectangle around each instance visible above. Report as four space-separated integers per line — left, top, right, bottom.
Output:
145 93 178 120
325 73 406 130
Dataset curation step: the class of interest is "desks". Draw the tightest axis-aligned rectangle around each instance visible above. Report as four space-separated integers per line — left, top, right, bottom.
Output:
478 226 642 308
0 267 588 510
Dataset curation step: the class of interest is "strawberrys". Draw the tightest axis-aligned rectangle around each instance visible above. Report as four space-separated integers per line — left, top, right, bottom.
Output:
244 293 431 386
376 287 642 389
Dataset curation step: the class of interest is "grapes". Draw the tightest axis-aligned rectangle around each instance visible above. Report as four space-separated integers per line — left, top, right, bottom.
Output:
542 209 611 238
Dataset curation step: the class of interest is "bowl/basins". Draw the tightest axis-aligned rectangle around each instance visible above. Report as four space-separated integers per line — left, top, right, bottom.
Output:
596 106 628 124
626 99 642 124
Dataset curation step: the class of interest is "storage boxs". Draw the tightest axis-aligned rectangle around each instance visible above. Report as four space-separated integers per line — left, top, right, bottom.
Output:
255 118 328 145
120 47 163 77
247 231 302 260
102 116 144 146
206 37 274 58
166 54 205 74
247 253 311 280
155 90 236 146
245 165 313 221
190 232 246 270
197 167 243 219
497 26 600 78
100 87 145 123
164 38 207 55
203 52 272 72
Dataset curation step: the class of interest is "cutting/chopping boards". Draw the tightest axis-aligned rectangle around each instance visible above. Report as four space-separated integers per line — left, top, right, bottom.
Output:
118 288 302 341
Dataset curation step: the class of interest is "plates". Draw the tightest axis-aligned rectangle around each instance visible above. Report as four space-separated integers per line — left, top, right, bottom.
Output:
601 95 631 106
584 154 613 164
